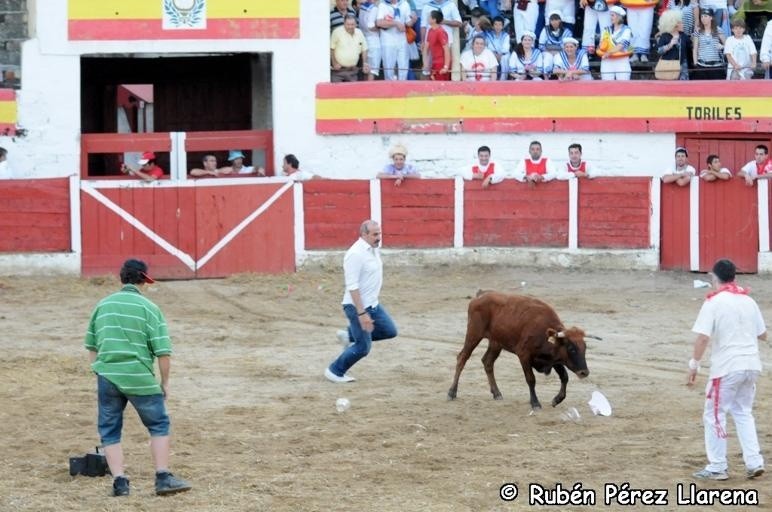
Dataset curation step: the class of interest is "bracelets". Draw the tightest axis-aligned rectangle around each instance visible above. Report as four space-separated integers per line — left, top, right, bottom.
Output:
688 359 699 369
357 311 368 316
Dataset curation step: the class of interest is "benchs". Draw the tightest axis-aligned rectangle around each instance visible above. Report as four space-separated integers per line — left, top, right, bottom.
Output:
332 14 771 82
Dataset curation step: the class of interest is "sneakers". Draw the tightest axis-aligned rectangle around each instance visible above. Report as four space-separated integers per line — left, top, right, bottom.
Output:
154 473 194 494
113 476 130 497
324 367 356 384
746 465 765 479
690 467 728 480
336 329 353 350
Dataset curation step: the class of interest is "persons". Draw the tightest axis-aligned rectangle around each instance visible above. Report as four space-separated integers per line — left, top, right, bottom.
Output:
121 152 164 181
687 258 767 480
658 145 772 187
324 218 398 384
189 154 222 177
555 144 599 181
281 154 321 182
461 146 504 187
512 141 554 185
84 258 192 496
375 145 422 186
218 150 266 176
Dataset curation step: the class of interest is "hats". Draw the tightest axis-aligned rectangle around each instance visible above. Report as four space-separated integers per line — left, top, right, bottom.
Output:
227 148 247 162
123 260 155 285
520 6 714 45
137 152 156 166
390 146 410 158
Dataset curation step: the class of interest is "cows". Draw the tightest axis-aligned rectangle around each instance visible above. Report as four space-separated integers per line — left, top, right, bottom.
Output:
447 289 603 412
